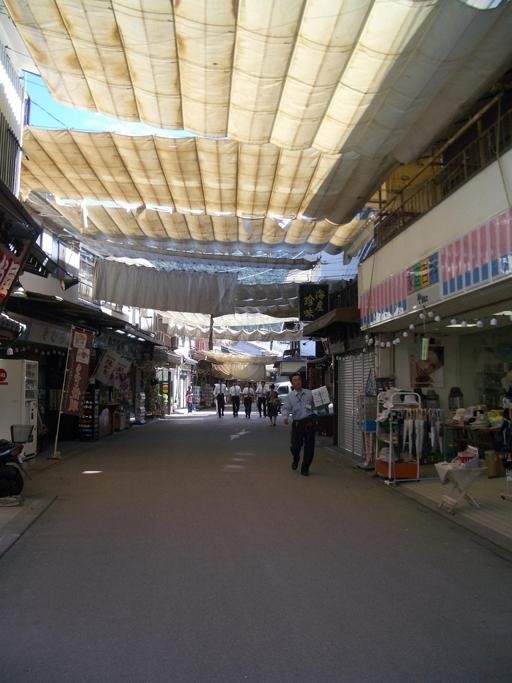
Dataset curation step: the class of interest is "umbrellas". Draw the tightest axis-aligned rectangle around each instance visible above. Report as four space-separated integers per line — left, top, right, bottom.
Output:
396 406 444 461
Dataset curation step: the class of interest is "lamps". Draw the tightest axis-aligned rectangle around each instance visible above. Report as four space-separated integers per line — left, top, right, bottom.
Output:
33 241 80 291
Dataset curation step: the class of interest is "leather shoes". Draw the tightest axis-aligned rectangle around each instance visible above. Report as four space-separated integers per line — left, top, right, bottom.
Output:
292 457 308 476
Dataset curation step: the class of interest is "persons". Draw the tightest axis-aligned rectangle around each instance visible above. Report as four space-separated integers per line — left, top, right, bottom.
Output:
409 345 445 385
186 384 193 413
243 381 255 418
229 381 243 417
266 383 280 426
212 378 228 418
254 381 267 417
281 371 320 477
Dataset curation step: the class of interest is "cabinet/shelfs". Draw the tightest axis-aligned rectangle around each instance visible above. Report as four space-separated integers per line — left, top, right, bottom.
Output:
358 395 376 433
375 391 421 482
77 389 100 441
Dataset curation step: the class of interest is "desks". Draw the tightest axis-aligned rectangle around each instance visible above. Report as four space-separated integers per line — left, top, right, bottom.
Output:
442 424 501 463
435 463 489 515
